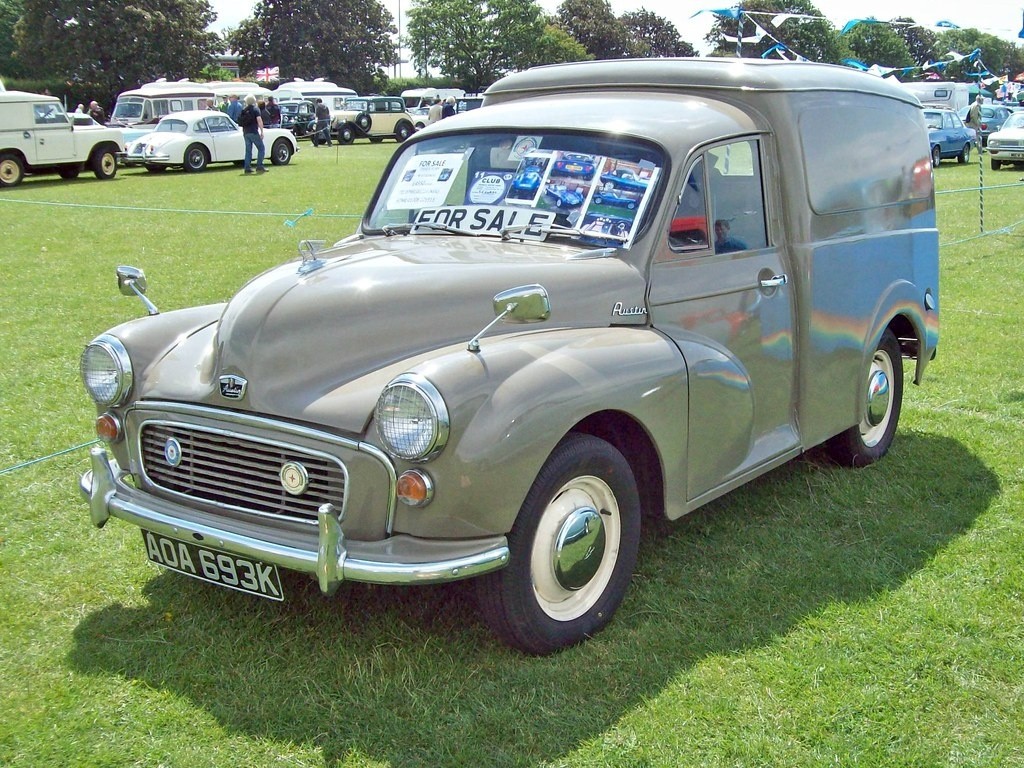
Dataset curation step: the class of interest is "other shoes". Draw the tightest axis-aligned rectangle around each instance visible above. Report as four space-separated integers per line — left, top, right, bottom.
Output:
982 150 988 153
256 167 269 172
245 169 254 173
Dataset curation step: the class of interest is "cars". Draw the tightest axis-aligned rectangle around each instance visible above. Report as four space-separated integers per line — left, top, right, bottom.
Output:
986 110 1024 171
0 87 125 189
593 192 638 210
76 54 944 657
957 103 1015 145
120 109 300 175
544 183 584 209
278 100 317 135
922 108 978 168
601 167 649 193
305 95 415 144
551 154 596 182
512 164 543 192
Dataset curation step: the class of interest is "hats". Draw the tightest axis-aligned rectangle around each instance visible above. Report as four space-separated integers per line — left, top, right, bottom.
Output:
976 94 985 99
243 95 256 105
446 96 455 105
223 94 228 98
230 95 237 99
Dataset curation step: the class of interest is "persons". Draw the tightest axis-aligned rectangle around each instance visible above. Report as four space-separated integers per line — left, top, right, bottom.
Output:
965 94 987 153
74 101 105 125
205 94 281 128
428 96 455 124
238 94 269 174
713 220 749 255
314 98 333 147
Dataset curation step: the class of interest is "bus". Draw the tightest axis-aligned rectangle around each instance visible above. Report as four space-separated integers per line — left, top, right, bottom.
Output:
398 87 468 133
109 77 279 121
276 80 359 133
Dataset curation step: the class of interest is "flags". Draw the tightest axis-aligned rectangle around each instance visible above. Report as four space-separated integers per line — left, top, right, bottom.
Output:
255 66 279 82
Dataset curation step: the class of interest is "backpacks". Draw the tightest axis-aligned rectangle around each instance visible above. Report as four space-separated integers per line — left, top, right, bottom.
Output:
965 109 970 123
237 107 254 127
